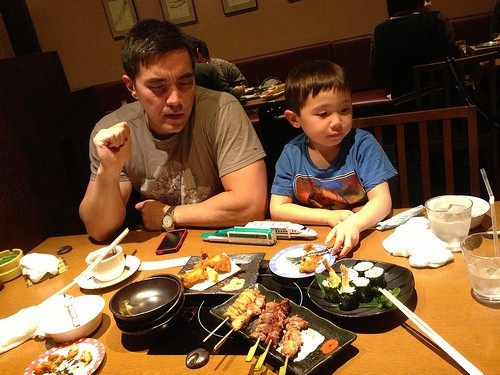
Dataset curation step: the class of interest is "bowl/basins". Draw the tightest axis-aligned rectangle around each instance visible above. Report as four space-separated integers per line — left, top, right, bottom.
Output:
109 273 185 338
43 294 105 343
427 195 491 229
85 244 125 282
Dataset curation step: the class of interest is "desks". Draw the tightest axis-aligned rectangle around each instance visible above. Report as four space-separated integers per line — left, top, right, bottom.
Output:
242 95 287 144
1 201 500 375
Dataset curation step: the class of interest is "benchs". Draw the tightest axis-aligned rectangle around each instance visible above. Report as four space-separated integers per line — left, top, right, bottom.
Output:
93 12 495 126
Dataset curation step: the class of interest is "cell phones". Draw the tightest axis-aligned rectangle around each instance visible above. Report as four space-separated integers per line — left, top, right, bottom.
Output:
156 229 188 255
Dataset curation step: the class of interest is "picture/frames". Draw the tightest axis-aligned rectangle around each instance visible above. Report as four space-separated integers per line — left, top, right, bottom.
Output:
159 0 197 26
221 0 258 15
101 0 140 40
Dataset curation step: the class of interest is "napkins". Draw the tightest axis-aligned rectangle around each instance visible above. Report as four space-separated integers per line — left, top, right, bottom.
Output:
0 295 71 355
19 253 60 283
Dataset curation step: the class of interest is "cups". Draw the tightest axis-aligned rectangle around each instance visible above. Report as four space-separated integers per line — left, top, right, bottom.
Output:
459 231 500 305
424 194 473 253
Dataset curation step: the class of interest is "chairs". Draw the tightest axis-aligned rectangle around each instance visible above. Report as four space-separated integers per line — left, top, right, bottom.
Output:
413 50 500 201
353 104 480 208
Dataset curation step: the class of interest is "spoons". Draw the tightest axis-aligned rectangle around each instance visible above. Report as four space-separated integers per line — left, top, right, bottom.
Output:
185 347 220 370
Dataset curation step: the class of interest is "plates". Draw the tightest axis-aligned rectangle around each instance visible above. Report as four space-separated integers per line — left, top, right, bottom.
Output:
23 338 106 375
209 282 357 375
268 243 335 278
176 252 266 296
307 257 415 318
260 91 283 98
75 254 141 290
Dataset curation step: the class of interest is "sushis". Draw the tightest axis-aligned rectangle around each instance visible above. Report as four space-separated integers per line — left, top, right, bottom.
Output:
321 261 386 310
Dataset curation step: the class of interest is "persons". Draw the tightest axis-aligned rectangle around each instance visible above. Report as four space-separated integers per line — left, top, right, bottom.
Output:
488 0 500 41
189 35 244 101
202 40 247 86
79 18 269 243
369 0 459 127
269 58 398 260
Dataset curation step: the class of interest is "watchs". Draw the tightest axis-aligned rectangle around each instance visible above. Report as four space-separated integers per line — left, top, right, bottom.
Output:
163 206 175 231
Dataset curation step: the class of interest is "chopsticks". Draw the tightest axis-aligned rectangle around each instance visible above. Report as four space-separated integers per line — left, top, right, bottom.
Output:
80 227 130 277
378 287 484 375
